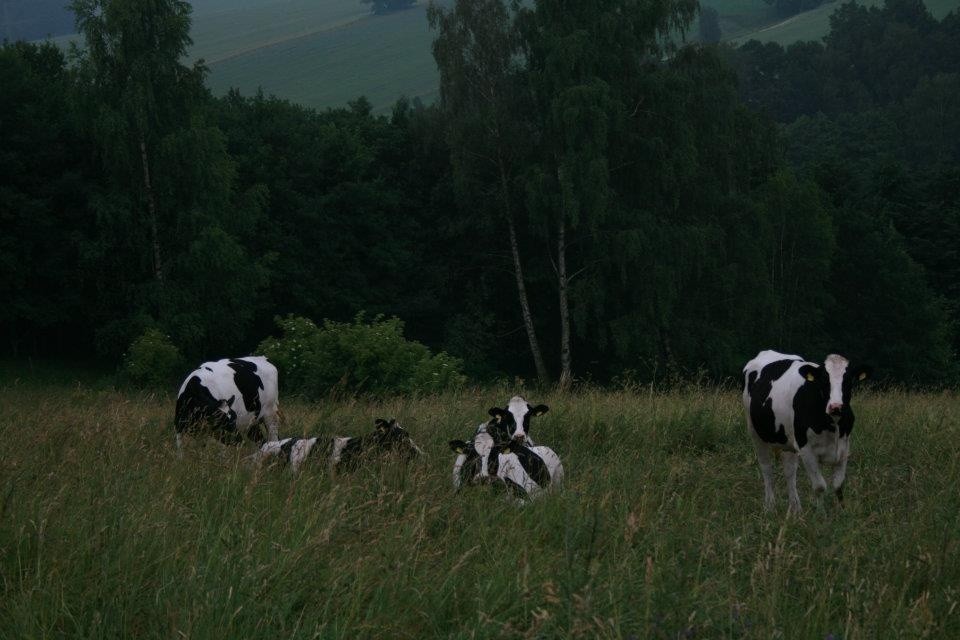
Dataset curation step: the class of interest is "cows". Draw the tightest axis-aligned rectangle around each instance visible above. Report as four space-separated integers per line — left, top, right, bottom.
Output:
237 417 432 478
742 349 856 519
450 396 565 503
174 355 279 444
